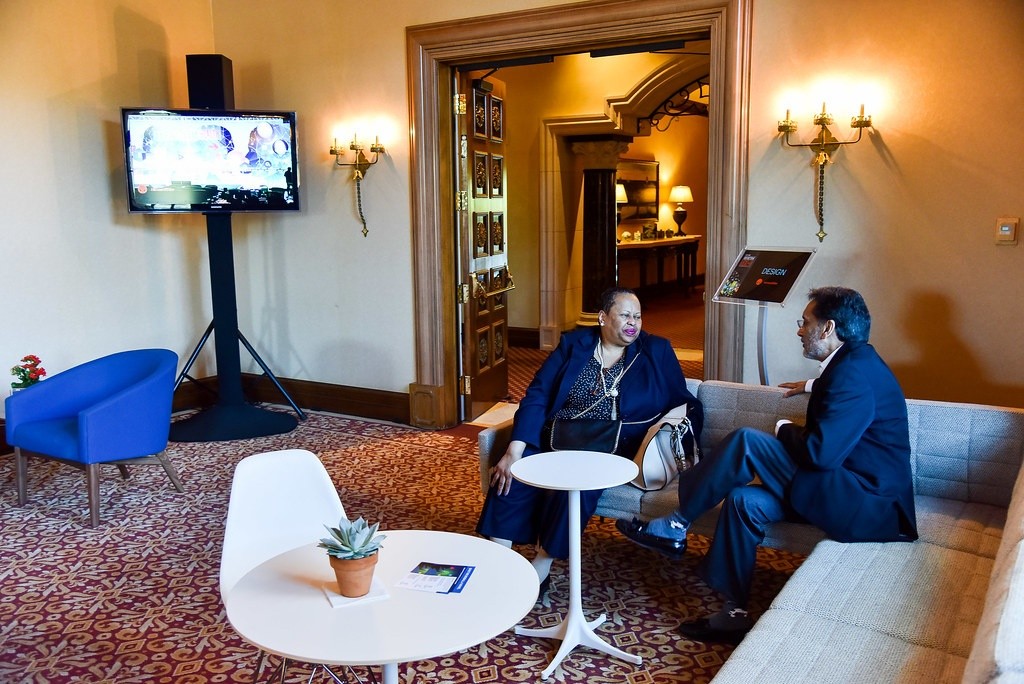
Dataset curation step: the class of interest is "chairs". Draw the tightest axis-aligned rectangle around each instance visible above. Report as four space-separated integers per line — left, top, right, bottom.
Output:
219 447 354 684
4 341 183 527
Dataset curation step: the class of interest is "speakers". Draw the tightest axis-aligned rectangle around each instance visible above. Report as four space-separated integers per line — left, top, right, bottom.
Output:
186 53 235 110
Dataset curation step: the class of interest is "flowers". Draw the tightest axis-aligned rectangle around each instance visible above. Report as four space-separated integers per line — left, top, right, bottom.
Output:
12 352 46 388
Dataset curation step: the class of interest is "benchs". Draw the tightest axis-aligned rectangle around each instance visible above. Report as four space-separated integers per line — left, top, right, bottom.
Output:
476 375 1024 684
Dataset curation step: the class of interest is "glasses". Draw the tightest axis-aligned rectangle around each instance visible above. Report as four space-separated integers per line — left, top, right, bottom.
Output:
797 318 837 330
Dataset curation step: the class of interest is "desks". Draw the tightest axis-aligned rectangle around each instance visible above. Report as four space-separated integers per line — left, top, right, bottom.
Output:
618 233 704 311
513 450 644 680
225 529 536 684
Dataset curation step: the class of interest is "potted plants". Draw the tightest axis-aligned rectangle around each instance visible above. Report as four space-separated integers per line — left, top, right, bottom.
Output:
314 512 388 603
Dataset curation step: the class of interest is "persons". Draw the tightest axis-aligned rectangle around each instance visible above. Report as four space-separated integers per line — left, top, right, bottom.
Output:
614 287 919 646
475 287 689 595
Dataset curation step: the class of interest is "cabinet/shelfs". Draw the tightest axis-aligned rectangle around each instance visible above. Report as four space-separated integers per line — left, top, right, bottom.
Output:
617 159 662 224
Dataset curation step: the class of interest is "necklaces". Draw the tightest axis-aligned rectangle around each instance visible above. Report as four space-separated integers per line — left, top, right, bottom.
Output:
598 338 625 420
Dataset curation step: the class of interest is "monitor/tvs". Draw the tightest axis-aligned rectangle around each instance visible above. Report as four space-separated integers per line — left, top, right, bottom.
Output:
119 106 303 213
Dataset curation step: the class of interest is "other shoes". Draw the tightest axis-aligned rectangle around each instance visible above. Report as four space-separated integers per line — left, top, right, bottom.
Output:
539 574 550 596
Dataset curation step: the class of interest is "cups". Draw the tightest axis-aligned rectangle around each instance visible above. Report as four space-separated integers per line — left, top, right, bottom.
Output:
666 229 674 238
633 232 641 242
657 229 664 239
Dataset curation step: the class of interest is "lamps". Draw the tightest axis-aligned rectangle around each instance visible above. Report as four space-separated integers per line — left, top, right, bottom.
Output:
778 89 871 250
327 133 389 236
668 183 695 238
617 183 628 242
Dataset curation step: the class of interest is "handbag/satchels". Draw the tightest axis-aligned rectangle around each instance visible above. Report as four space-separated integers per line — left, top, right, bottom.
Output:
630 402 699 491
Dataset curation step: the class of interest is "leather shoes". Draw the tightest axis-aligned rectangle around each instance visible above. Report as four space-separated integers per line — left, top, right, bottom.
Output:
679 619 753 646
615 518 687 560
